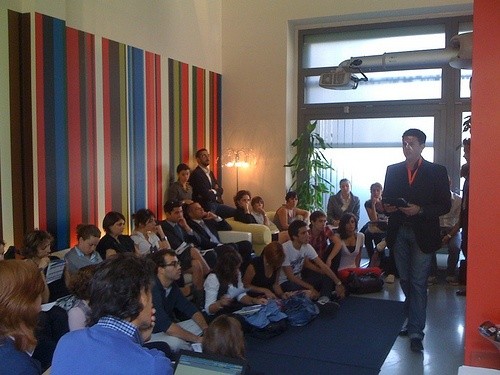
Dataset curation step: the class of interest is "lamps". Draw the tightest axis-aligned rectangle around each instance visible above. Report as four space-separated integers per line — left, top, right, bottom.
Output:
214 144 258 194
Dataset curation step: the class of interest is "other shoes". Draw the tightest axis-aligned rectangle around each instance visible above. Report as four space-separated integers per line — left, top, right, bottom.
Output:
445 275 461 286
398 326 409 337
426 274 439 286
455 287 466 296
410 338 424 355
316 300 341 315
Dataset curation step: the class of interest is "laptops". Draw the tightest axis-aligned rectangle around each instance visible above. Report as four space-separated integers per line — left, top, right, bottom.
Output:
172 349 248 375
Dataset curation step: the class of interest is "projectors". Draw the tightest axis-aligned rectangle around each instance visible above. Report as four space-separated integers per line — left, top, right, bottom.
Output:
319 71 355 90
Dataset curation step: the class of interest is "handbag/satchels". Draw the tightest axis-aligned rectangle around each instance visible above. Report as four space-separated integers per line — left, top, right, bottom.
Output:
284 292 320 327
350 272 384 294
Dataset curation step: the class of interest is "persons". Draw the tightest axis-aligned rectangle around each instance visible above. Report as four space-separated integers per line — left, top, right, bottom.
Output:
432 174 462 285
0 151 396 375
456 138 470 296
382 129 452 349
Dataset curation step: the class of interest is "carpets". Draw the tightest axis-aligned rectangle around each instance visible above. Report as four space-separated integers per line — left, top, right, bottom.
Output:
245 296 407 375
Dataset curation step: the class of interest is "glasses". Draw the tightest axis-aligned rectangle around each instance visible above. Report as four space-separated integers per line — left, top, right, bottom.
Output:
158 260 183 268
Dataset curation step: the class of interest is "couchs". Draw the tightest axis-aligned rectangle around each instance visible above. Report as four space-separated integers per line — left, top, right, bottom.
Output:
225 211 311 256
49 231 252 301
